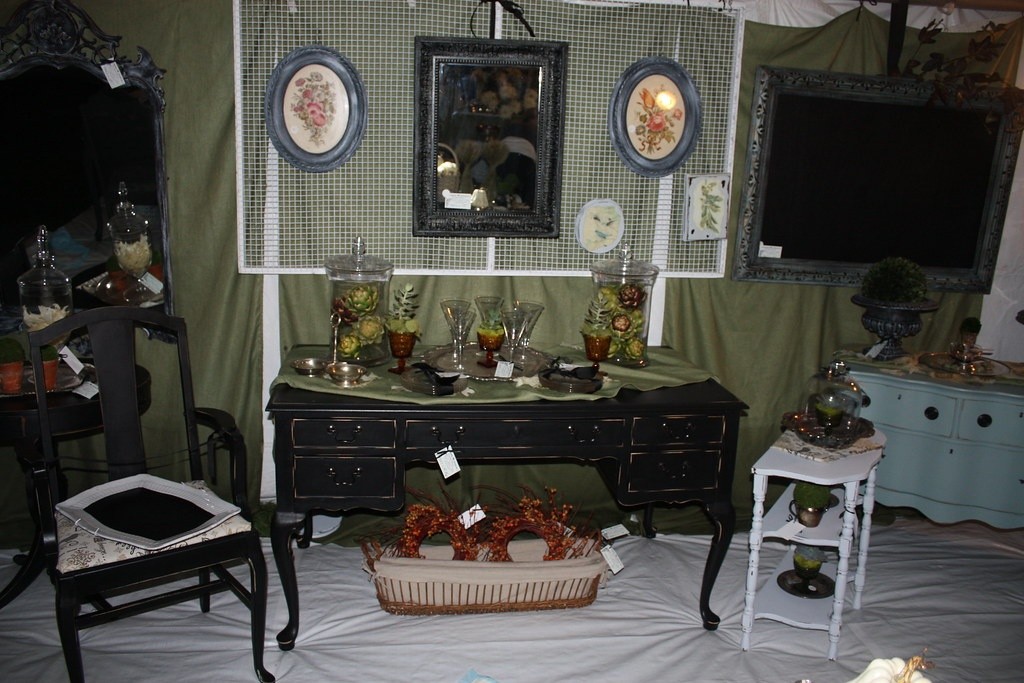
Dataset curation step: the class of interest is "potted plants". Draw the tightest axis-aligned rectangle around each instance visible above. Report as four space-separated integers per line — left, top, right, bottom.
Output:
848 255 939 359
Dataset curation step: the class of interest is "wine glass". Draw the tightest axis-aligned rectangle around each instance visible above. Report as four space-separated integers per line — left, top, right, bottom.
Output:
439 298 472 362
512 298 546 364
474 296 505 357
442 305 477 373
499 307 530 363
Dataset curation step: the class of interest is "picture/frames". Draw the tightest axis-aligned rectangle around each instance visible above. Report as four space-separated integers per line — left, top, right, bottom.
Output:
265 45 367 175
606 56 704 178
729 63 1024 296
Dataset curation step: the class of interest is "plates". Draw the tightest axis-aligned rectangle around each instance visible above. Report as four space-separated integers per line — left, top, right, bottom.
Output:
265 45 365 174
609 55 702 178
400 367 469 395
574 198 625 253
538 368 603 394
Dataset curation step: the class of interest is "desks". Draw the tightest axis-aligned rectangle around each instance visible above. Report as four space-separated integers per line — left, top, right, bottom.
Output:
0 353 152 611
262 342 750 651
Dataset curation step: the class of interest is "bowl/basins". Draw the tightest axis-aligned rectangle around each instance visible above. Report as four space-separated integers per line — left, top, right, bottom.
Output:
324 364 367 382
288 357 328 375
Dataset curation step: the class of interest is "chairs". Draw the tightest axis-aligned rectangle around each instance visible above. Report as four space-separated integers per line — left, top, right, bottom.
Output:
27 304 275 683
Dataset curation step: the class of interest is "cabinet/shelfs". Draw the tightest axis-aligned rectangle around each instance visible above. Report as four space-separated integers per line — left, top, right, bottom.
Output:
838 352 1024 530
743 429 882 663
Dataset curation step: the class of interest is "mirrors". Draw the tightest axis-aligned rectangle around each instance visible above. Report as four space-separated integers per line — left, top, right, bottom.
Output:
0 0 176 343
411 34 568 239
